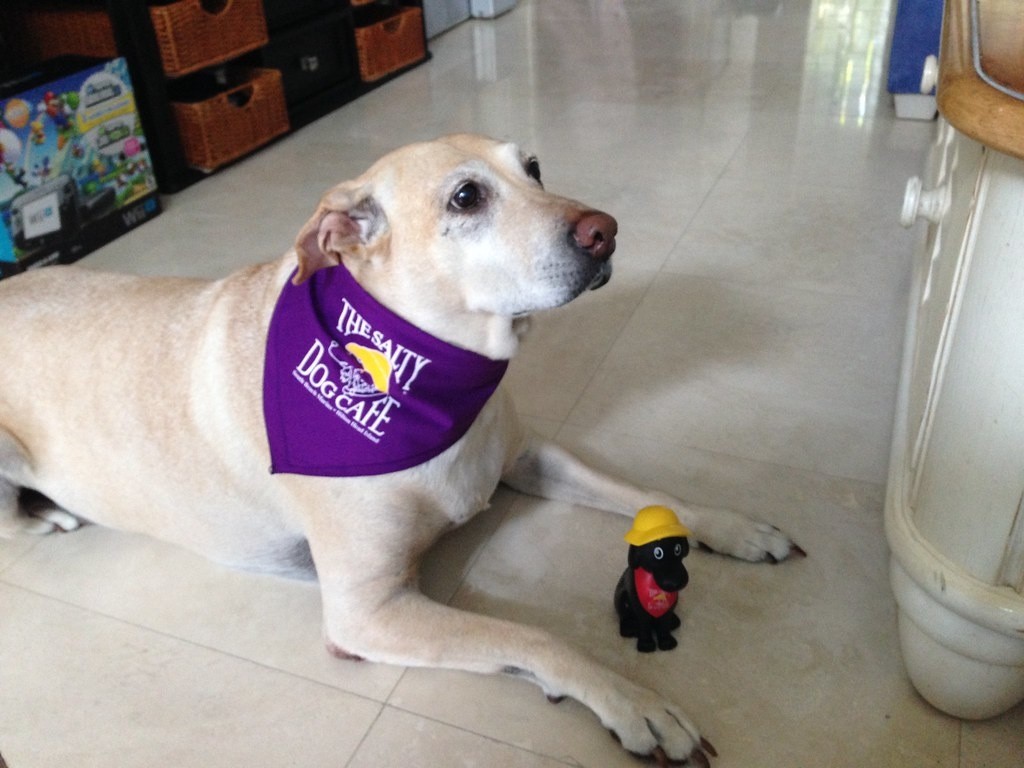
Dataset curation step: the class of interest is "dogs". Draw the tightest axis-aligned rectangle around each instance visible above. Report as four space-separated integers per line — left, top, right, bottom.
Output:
0 133 808 768
613 506 693 653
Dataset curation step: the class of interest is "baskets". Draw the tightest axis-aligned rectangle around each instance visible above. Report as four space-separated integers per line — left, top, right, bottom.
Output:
354 6 426 82
26 0 269 78
173 67 291 169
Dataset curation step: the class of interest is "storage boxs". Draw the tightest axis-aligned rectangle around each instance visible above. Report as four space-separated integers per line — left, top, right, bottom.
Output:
264 0 347 32
272 9 355 105
0 53 157 262
